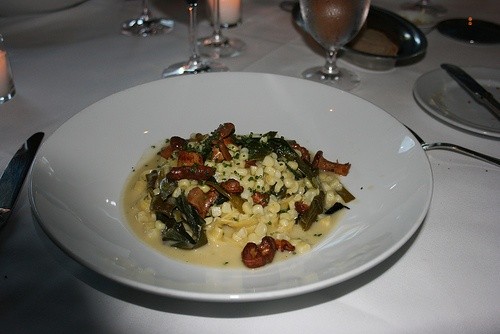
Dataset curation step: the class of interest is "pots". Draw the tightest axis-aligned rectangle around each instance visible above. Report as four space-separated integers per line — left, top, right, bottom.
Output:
279 0 428 70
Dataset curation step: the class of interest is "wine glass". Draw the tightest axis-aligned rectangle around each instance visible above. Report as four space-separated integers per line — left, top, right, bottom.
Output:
301 0 365 93
161 0 230 77
120 0 173 43
193 0 246 60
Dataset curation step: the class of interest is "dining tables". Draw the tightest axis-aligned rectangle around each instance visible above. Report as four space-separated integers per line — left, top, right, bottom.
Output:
0 0 500 334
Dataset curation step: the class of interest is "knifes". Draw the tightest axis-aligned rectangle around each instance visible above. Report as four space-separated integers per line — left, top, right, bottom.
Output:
440 63 500 117
0 131 46 231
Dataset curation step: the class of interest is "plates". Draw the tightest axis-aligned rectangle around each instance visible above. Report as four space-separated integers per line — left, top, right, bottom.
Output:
29 71 434 302
436 18 500 44
411 64 500 138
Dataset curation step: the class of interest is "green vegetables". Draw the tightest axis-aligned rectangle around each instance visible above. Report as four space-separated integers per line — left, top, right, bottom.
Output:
234 130 320 190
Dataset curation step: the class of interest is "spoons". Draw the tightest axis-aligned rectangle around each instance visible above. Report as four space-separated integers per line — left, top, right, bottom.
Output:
401 124 500 167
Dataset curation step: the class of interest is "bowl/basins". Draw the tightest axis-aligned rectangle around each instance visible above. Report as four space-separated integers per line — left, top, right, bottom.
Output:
395 10 436 36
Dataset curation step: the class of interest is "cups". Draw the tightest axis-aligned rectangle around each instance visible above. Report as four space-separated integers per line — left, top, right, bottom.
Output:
0 34 15 104
207 0 241 28
